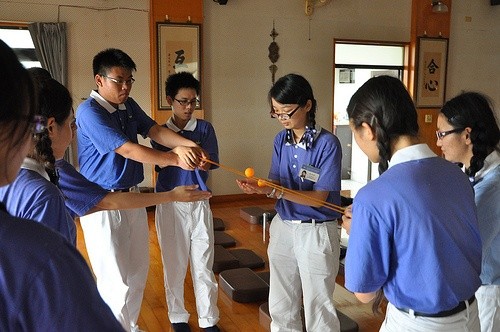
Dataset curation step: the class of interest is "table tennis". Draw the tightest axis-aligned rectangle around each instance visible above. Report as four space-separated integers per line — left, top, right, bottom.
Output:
245 167 255 178
257 180 266 187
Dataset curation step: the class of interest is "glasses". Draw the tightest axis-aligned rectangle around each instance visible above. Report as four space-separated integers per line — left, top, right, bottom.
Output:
172 98 200 105
23 114 47 134
101 75 135 84
436 128 463 139
269 105 301 120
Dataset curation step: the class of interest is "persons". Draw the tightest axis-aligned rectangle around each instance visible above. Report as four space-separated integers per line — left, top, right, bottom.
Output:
234 71 343 331
2 75 78 246
148 70 223 332
340 73 484 331
24 66 213 250
0 37 128 332
73 48 213 332
435 93 500 331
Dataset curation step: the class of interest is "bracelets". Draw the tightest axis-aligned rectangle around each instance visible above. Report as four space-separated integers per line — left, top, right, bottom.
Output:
276 188 285 200
266 184 275 197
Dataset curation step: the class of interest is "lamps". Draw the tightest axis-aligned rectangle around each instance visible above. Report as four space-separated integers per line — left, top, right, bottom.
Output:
429 0 450 15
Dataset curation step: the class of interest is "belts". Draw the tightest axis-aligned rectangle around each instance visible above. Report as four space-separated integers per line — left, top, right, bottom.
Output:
301 219 336 224
396 295 476 317
108 186 138 192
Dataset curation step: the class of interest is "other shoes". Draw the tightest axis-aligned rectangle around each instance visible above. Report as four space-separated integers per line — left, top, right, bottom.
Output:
173 323 190 331
205 325 219 331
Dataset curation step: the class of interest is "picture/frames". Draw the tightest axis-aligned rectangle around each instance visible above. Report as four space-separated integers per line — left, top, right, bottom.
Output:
414 33 450 109
155 20 203 111
